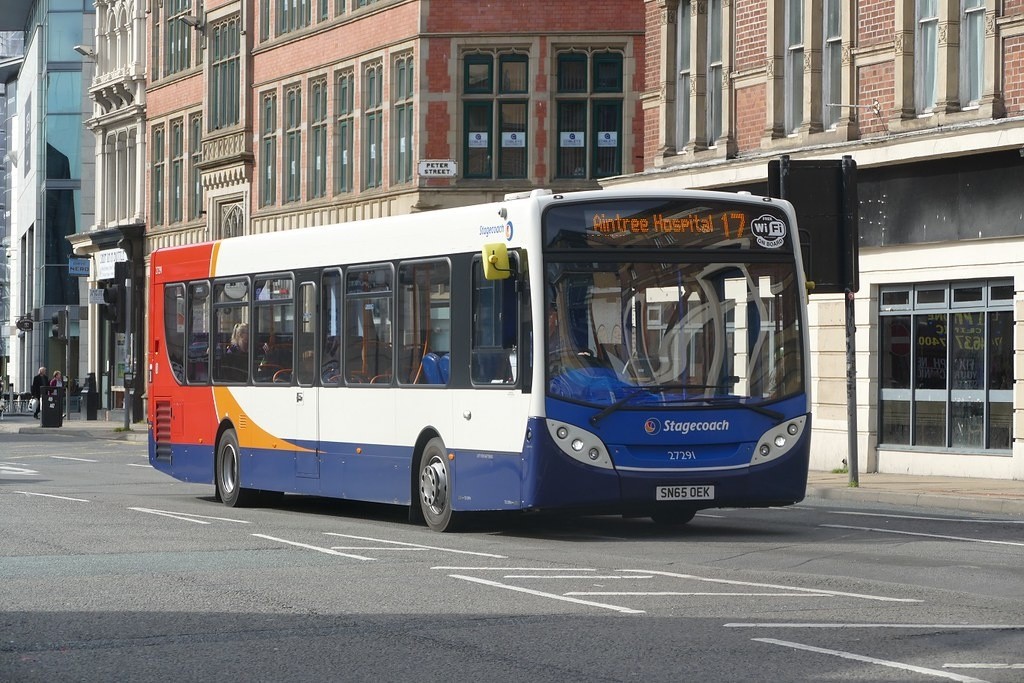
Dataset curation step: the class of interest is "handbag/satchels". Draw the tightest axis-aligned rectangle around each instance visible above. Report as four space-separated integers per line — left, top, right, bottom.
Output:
28 398 39 414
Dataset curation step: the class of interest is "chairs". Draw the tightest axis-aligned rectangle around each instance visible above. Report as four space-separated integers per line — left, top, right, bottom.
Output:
190 336 450 384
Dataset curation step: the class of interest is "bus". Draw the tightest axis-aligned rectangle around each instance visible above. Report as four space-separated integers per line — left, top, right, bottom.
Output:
146 191 813 532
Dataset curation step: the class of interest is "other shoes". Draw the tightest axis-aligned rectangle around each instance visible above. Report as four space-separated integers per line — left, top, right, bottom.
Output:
33 415 40 419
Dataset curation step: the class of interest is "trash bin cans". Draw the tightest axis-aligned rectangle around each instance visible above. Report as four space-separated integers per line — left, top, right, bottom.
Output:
40 385 63 428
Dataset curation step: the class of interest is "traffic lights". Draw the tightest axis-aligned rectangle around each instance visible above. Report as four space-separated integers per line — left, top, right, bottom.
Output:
51 308 66 342
102 283 125 333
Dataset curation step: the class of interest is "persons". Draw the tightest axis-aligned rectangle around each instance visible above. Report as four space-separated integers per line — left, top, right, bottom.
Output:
225 323 270 379
48 371 66 417
529 307 578 367
32 367 50 419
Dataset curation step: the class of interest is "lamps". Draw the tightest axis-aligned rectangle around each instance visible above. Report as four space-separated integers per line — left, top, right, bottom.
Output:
179 15 205 36
73 45 98 64
